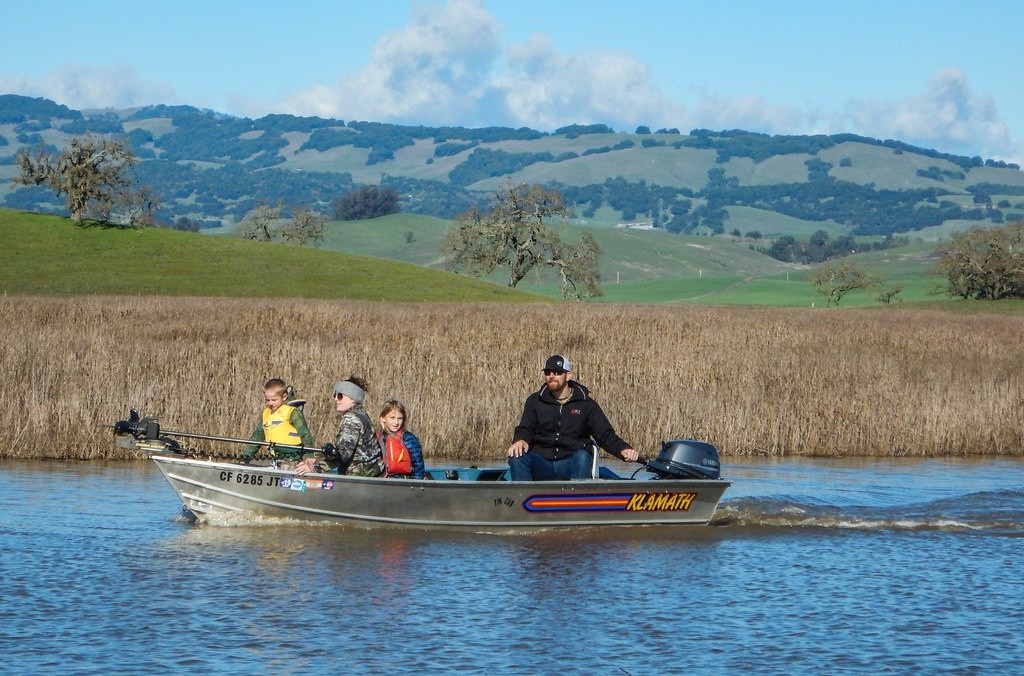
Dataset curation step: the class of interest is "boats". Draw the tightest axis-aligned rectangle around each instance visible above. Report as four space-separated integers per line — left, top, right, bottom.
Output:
102 407 733 526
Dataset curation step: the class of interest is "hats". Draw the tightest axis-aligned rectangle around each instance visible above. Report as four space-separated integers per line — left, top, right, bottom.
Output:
541 355 570 372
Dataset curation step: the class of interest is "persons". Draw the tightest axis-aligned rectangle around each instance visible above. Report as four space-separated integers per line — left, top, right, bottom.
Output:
507 354 640 480
375 399 425 479
237 378 315 470
295 375 384 477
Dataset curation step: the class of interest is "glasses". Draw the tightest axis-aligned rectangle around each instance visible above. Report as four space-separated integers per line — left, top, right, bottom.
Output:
333 391 343 400
544 370 566 376
